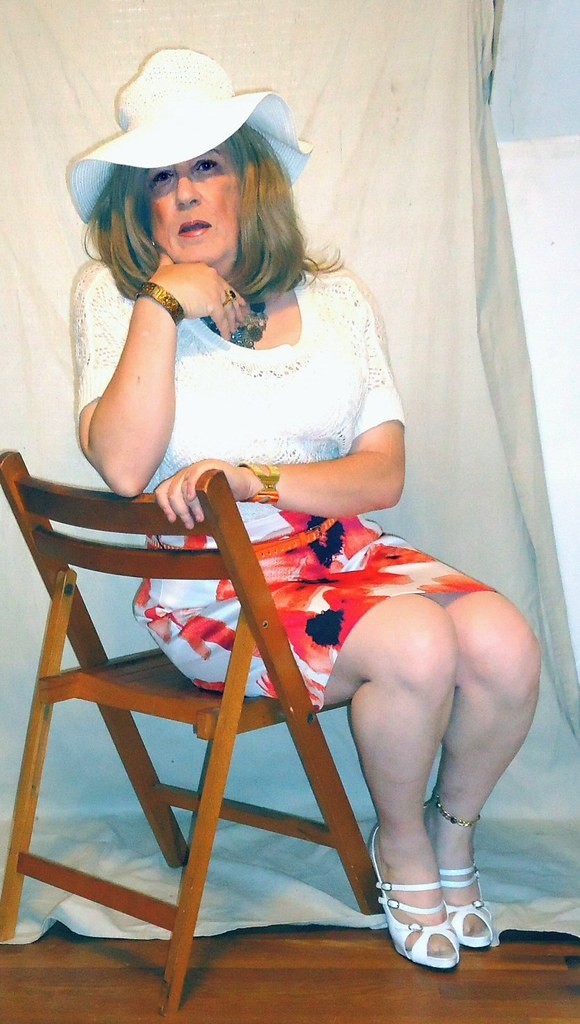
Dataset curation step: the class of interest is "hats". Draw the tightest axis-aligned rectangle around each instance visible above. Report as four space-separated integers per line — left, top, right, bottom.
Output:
69 50 315 224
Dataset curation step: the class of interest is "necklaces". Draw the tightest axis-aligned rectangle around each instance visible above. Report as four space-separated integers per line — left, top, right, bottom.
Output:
200 291 269 351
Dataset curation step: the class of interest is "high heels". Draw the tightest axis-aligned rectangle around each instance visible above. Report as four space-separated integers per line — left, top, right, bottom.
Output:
370 826 459 969
439 863 493 948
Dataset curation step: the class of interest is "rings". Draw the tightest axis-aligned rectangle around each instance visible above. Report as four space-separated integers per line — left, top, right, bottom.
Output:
222 289 237 307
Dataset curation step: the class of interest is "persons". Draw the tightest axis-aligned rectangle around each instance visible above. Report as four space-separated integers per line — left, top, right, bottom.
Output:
68 49 541 970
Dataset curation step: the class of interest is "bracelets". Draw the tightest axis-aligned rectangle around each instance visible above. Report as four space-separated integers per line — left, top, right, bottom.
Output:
238 463 280 506
135 282 185 326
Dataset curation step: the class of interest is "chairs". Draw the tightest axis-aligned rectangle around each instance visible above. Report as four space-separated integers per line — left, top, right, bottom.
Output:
0 451 385 1019
